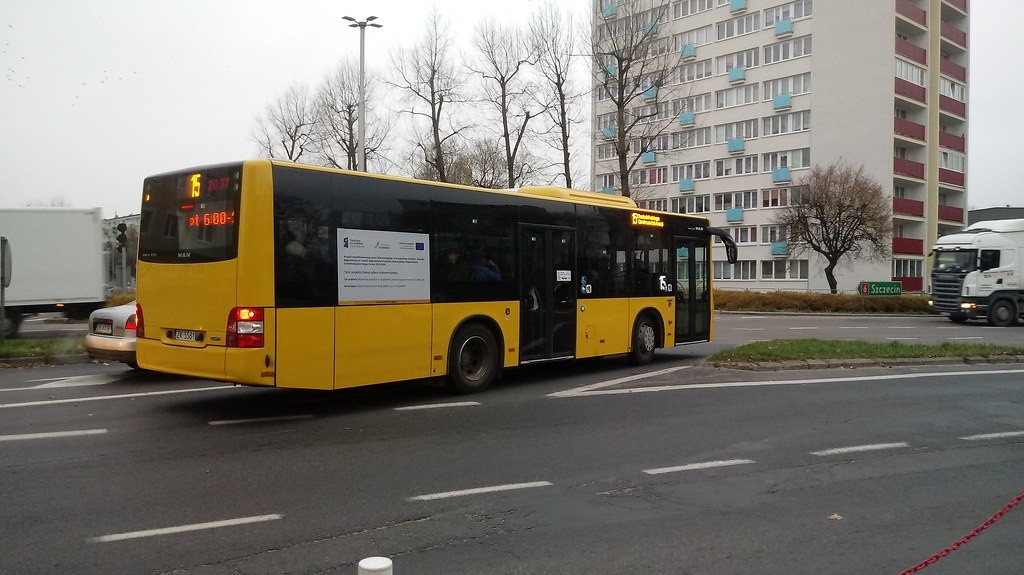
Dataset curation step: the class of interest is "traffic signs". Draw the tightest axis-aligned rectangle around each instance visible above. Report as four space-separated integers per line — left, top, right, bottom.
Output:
856 281 903 297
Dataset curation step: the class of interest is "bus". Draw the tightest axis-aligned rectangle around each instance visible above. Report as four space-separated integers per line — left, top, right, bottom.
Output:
136 160 739 395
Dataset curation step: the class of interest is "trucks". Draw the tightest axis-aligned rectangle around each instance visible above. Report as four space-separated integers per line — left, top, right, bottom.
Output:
0 206 108 336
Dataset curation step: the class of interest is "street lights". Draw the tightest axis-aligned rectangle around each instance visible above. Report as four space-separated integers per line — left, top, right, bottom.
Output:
342 15 383 173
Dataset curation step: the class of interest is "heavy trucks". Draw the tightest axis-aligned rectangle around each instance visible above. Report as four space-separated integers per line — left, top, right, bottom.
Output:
923 218 1024 329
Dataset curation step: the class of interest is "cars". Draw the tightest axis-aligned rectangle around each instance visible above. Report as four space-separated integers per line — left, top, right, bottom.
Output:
84 298 142 373
